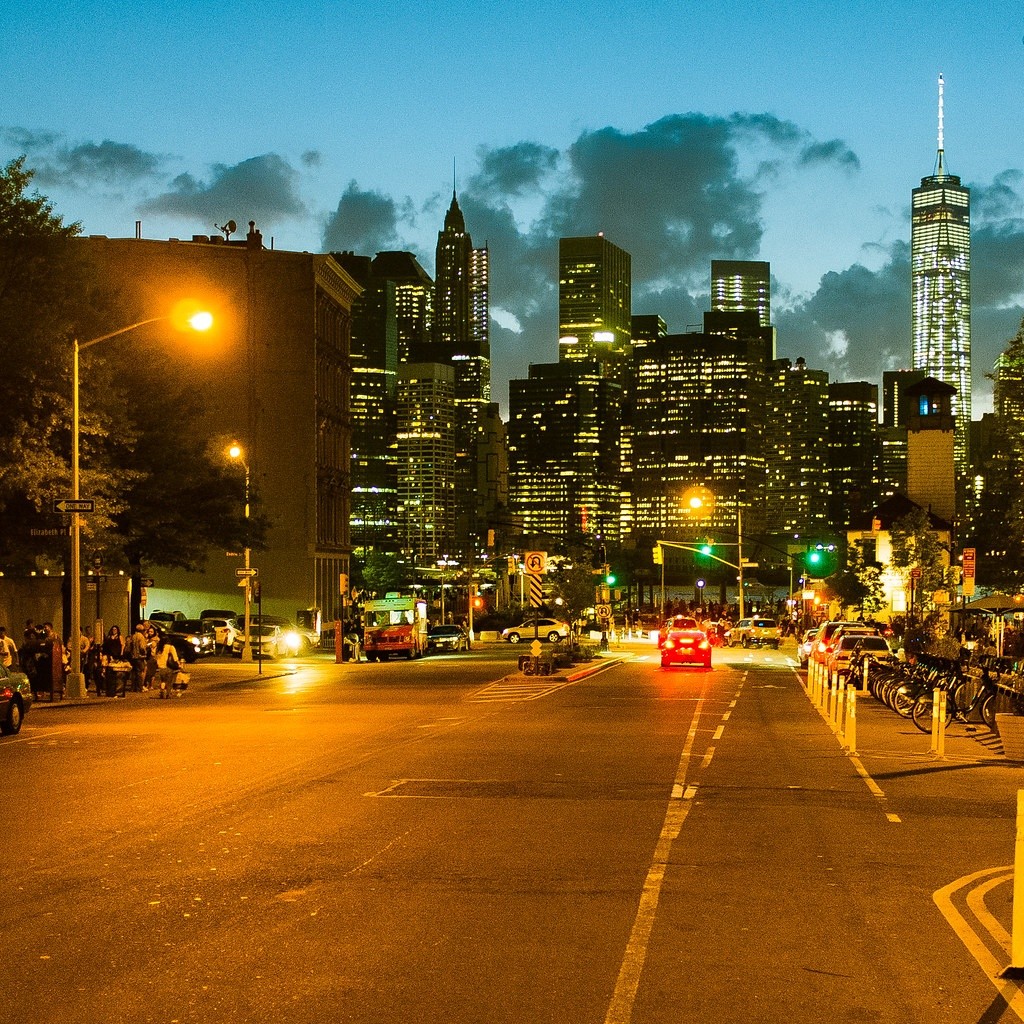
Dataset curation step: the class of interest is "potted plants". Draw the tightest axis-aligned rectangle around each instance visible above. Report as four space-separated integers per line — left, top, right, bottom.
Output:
995 674 1024 762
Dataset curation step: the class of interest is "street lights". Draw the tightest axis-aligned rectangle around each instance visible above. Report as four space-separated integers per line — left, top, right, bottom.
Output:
692 498 743 618
93 551 105 696
66 304 212 702
0 661 33 734
468 549 520 649
230 447 253 663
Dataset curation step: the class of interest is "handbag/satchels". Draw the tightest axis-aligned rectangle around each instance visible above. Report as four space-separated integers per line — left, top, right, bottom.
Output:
167 647 179 670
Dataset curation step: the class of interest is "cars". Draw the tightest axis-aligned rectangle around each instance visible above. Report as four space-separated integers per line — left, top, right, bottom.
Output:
658 617 711 668
502 617 570 643
797 628 819 668
828 635 893 689
428 624 469 654
233 625 289 660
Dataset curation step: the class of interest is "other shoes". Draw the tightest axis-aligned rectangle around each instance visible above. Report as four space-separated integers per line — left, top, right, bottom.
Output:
167 695 171 698
141 686 148 692
160 692 164 698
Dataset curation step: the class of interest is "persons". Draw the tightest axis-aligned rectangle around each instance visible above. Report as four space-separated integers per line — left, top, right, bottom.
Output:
821 612 872 621
0 621 180 699
668 597 729 620
780 608 797 637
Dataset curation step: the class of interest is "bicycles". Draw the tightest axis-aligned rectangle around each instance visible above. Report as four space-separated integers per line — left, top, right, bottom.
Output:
838 653 1020 734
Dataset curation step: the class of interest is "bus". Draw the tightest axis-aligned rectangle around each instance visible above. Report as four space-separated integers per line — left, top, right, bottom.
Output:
364 592 428 660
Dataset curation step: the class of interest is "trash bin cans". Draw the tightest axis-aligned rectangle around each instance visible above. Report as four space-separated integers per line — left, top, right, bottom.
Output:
106 666 131 698
518 655 530 671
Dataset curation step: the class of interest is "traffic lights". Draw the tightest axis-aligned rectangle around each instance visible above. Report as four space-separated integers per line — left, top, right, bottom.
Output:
703 538 713 558
606 575 616 587
472 598 483 609
811 552 821 566
652 547 663 564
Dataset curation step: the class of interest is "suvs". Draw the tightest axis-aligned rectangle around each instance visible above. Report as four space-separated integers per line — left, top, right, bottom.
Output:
234 614 320 657
811 621 882 663
162 610 237 661
724 617 781 650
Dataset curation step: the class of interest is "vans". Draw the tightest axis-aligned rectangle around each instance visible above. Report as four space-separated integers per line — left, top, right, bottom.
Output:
149 610 187 633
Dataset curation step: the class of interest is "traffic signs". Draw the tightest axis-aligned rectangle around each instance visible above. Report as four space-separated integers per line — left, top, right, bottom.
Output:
54 499 95 513
